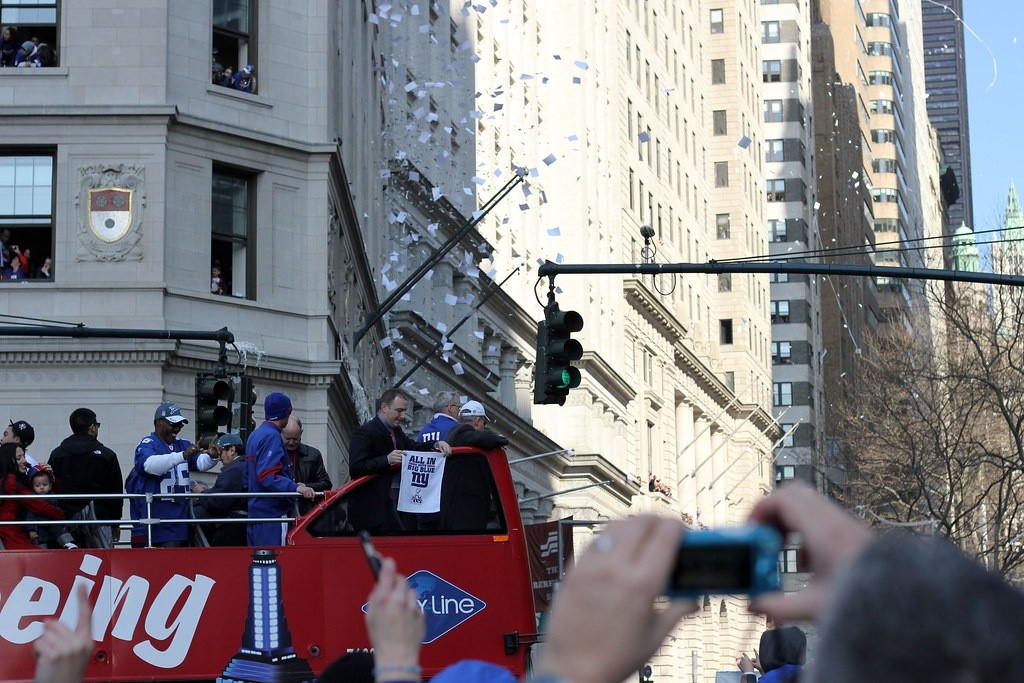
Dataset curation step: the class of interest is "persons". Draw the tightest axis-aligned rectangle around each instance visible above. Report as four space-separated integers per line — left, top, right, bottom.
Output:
124 392 333 548
0 28 57 67
0 408 123 549
349 387 508 537
35 482 1024 683
211 260 224 295
0 228 52 280
212 48 256 94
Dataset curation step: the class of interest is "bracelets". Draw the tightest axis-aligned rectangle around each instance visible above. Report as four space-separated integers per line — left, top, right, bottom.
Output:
435 441 439 450
372 664 422 674
296 485 301 492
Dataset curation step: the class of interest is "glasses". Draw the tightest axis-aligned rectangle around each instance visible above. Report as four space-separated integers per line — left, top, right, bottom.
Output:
446 403 462 410
163 419 185 429
90 422 101 428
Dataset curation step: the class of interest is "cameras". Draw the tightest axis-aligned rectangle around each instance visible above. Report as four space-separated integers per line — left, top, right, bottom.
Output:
663 526 783 598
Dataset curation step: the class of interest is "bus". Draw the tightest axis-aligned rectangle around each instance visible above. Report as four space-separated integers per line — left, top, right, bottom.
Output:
0 445 537 683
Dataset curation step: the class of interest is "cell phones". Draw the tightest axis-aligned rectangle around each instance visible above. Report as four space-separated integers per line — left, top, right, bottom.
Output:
359 530 382 581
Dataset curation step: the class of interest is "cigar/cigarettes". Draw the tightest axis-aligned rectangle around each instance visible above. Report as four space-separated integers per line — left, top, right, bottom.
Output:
194 481 198 484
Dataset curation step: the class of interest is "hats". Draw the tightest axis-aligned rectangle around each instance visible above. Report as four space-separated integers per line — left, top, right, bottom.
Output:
458 400 491 422
265 392 293 421
9 418 35 445
217 434 244 445
154 403 188 425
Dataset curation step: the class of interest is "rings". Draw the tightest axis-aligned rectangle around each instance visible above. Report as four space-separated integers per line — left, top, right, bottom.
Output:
596 534 615 552
192 447 195 450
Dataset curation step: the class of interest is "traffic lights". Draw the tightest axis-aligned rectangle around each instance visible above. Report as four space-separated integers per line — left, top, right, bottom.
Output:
534 311 583 406
194 377 230 449
